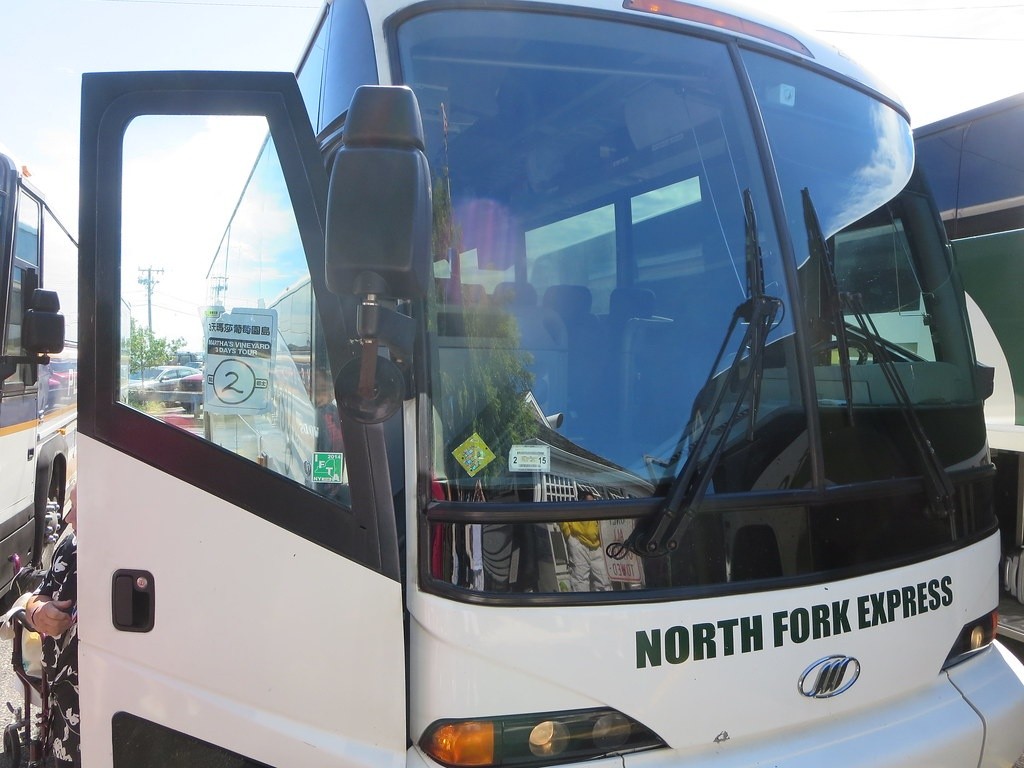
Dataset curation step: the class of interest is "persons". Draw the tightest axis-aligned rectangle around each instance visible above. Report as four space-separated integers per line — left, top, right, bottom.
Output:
26 482 81 768
556 490 614 592
433 143 557 345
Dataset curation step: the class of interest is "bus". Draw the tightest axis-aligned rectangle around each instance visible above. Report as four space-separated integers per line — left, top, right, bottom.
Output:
0 153 131 606
77 0 1024 768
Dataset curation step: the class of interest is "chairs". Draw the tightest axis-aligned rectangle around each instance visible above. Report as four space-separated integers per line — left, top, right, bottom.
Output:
437 277 673 423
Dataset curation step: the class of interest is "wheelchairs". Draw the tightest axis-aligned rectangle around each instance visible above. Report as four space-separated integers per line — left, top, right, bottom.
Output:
4 554 81 768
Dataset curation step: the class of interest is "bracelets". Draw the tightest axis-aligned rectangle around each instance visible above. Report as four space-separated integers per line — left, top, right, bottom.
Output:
31 606 41 626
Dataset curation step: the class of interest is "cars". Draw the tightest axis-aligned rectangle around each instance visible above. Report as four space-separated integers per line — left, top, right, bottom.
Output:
126 360 204 412
48 361 77 409
